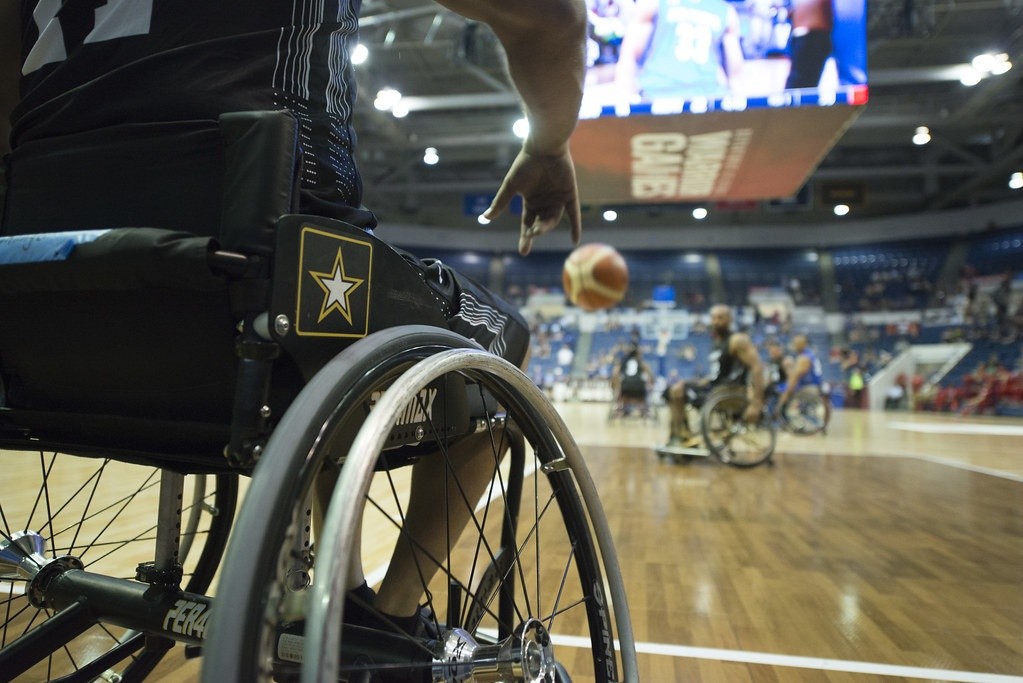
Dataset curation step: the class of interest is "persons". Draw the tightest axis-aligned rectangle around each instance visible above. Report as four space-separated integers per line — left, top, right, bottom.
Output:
492 268 1023 462
587 0 832 103
0 0 586 683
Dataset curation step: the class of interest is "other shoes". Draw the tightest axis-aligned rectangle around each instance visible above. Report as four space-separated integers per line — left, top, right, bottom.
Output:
343 594 433 621
341 614 446 659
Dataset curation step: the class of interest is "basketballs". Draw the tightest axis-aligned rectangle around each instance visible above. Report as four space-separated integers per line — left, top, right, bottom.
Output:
562 241 630 311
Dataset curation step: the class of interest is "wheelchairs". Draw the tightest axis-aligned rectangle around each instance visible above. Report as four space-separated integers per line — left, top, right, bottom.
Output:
0 107 640 683
654 382 832 469
